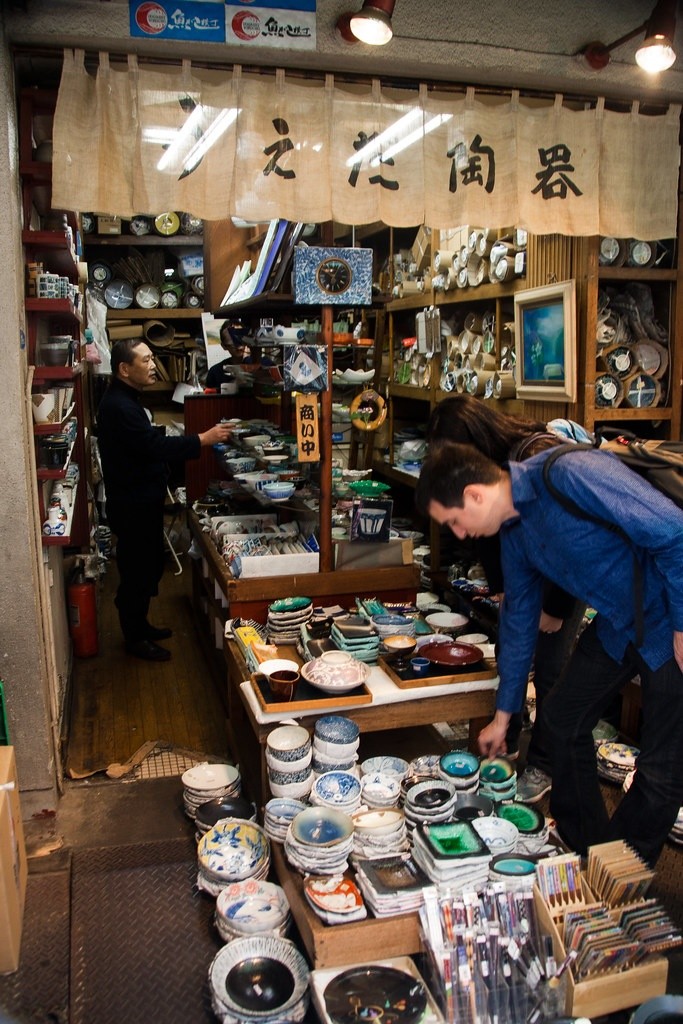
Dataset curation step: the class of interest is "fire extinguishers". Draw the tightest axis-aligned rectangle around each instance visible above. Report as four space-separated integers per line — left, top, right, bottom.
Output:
67 557 99 660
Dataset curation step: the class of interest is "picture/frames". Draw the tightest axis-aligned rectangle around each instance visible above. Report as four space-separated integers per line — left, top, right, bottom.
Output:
512 277 580 406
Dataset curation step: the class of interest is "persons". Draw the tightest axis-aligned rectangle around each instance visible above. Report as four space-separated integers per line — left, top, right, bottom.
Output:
205 322 283 390
418 445 683 876
94 338 242 658
427 396 607 801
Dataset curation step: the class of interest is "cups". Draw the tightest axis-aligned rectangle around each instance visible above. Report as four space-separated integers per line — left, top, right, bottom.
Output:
259 658 300 702
32 382 76 425
260 318 274 333
40 335 76 367
357 509 386 535
409 657 430 677
42 435 69 470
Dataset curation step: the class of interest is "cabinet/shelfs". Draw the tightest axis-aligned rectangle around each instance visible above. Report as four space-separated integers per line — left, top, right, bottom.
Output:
10 86 683 706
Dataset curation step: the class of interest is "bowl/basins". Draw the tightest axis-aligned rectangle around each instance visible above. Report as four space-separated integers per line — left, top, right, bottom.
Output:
213 419 305 503
417 611 489 644
333 333 353 346
300 649 370 694
284 715 518 873
181 723 313 1017
370 613 415 663
273 326 321 345
489 852 537 885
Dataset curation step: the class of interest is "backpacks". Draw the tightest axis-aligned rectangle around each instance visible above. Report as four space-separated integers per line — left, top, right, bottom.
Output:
543 431 682 647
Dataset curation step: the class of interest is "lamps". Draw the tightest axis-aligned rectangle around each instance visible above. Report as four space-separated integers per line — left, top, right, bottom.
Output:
157 90 245 176
345 84 467 170
576 1 678 73
335 0 397 47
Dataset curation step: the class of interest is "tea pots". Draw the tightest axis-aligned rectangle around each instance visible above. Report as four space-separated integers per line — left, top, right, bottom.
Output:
448 562 484 579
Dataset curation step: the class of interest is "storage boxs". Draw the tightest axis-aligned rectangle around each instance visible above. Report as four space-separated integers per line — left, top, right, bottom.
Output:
0 745 29 974
530 870 669 1018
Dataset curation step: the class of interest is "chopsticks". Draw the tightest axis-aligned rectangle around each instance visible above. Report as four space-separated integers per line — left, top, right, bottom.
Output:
442 838 682 1024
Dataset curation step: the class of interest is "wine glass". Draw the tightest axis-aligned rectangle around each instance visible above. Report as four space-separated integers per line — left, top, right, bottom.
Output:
384 635 417 671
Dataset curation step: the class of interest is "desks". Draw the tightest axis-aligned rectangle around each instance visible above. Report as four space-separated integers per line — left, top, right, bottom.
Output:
269 837 420 967
220 636 499 817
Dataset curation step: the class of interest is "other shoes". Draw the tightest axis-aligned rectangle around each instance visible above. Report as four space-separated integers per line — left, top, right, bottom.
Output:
140 627 173 639
124 638 171 662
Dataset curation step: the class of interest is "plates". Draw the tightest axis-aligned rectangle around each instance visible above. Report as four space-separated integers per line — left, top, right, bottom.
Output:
418 641 483 669
265 597 313 645
596 743 641 794
331 458 391 533
323 965 427 1024
590 233 660 407
303 876 363 913
355 819 493 918
496 801 545 834
335 368 376 384
82 211 207 307
